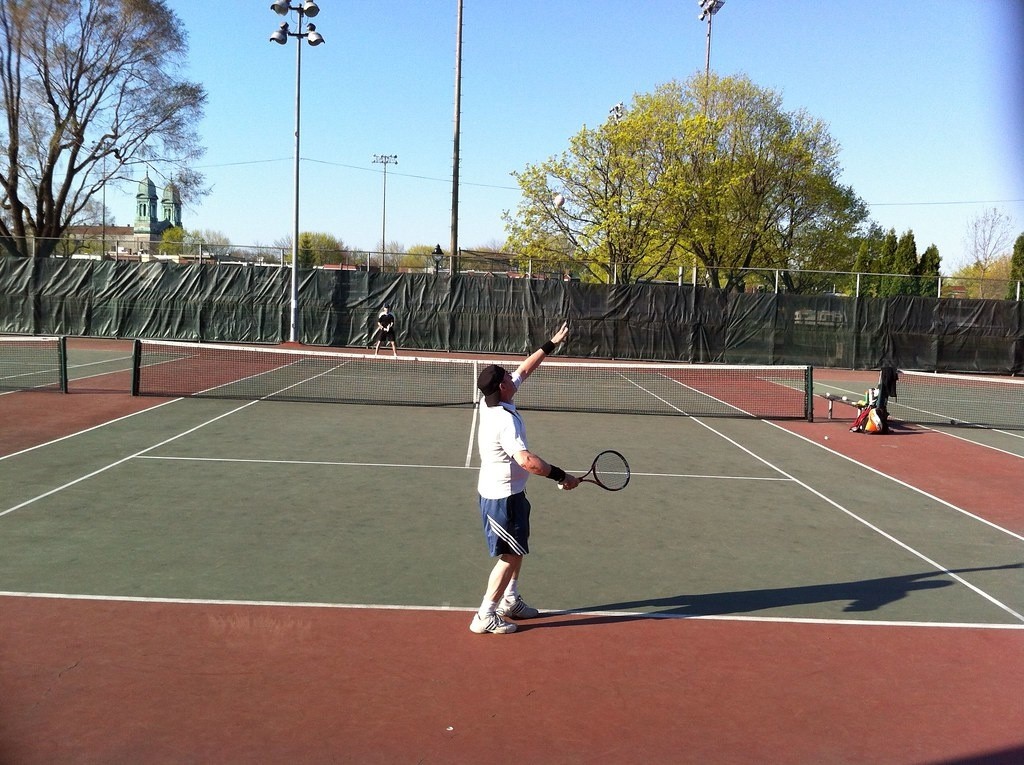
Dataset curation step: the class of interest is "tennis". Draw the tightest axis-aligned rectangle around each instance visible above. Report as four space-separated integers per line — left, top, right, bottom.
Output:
824 436 829 440
555 194 566 206
558 483 563 489
842 395 847 400
826 392 830 396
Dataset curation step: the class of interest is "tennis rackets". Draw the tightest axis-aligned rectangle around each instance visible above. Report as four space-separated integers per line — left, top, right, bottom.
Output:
557 450 630 491
386 324 390 332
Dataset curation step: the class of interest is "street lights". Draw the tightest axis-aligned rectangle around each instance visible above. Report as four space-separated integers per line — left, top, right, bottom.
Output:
696 0 726 120
371 153 398 273
268 0 326 349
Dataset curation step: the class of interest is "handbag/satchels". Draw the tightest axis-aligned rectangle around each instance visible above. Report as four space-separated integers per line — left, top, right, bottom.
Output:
849 405 884 434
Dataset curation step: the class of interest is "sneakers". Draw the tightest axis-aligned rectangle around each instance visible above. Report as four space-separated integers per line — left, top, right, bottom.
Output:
469 608 517 635
496 594 538 618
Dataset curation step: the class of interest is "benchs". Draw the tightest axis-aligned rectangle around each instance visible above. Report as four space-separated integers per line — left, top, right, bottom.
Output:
820 394 869 420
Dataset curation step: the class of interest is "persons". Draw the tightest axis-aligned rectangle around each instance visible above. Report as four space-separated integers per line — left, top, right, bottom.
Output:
469 321 581 635
374 303 398 356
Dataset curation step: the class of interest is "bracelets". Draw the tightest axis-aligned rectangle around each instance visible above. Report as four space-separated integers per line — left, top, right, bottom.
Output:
540 340 556 355
546 464 567 483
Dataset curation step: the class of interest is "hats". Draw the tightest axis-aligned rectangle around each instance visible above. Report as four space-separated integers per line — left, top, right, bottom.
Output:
477 364 505 407
383 303 389 308
